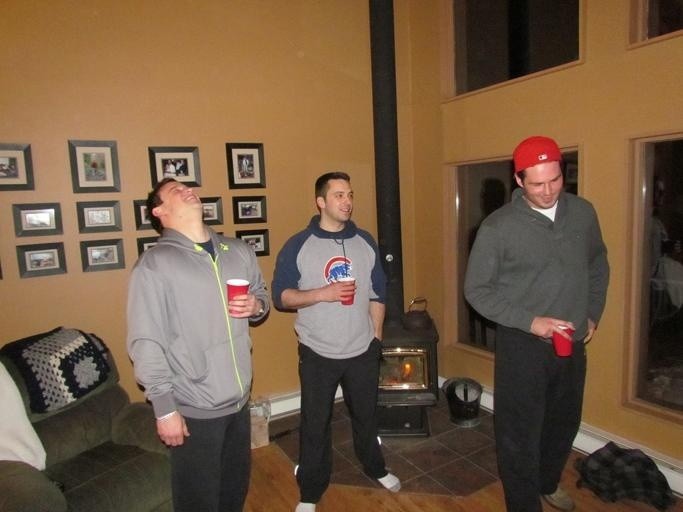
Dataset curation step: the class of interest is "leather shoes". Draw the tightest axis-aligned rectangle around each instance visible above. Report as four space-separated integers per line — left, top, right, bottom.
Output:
543 484 576 512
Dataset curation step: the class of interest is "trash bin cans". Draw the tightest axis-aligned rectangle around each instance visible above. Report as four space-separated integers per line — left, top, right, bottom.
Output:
249 401 271 449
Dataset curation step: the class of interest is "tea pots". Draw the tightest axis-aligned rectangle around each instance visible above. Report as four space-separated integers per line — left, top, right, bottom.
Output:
398 296 432 331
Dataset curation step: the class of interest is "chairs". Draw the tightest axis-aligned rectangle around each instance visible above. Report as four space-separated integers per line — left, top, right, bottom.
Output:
0 325 178 512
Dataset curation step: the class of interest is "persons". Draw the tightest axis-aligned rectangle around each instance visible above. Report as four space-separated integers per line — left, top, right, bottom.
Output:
241 158 249 177
649 175 681 330
175 160 186 176
125 178 271 511
272 171 401 511
461 136 609 511
163 160 175 177
90 159 97 177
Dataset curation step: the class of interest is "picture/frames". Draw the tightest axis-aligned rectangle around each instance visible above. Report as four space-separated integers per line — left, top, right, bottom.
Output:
67 138 121 193
0 199 127 280
0 142 35 191
134 142 270 256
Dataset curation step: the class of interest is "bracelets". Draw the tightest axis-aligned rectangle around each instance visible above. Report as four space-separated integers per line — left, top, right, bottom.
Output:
254 298 265 320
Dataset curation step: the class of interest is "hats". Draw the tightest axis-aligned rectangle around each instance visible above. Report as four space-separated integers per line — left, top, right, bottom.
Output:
511 135 564 172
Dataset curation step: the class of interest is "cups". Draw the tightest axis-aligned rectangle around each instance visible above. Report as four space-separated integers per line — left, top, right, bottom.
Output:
225 279 250 315
553 326 573 357
338 278 356 306
